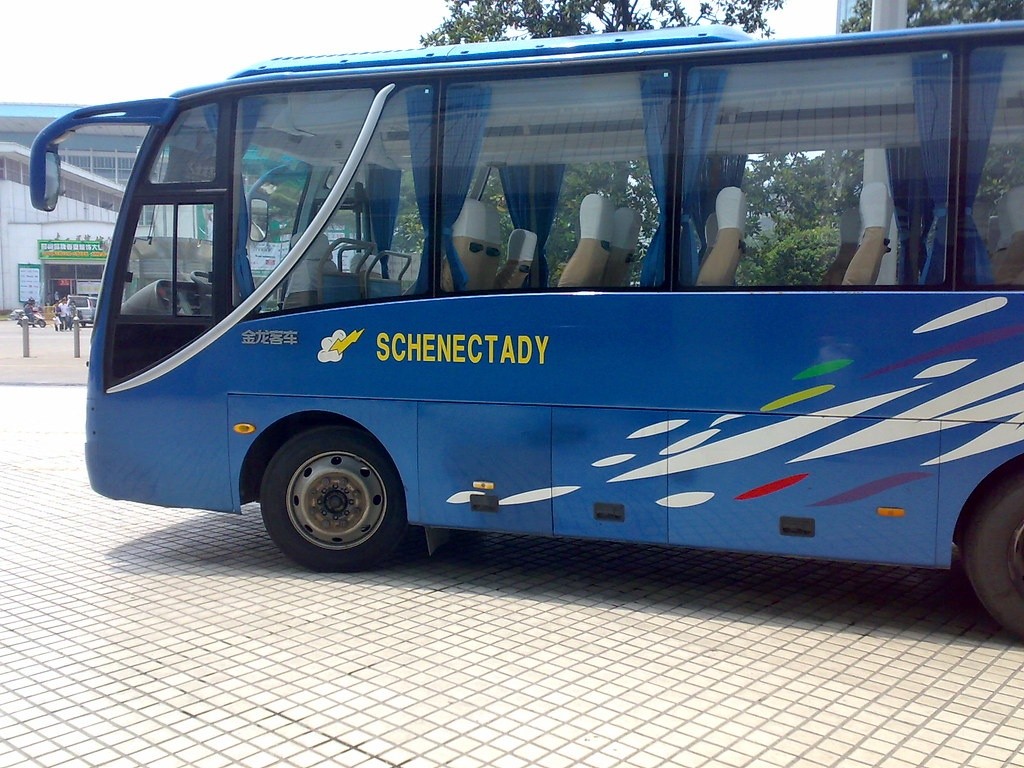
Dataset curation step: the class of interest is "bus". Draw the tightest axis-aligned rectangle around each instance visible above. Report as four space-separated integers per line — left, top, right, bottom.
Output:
27 15 1024 648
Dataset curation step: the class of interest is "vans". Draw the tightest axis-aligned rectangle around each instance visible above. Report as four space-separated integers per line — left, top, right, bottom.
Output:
64 294 97 329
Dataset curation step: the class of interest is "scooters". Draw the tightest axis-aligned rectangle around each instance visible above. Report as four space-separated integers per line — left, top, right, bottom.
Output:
16 305 46 328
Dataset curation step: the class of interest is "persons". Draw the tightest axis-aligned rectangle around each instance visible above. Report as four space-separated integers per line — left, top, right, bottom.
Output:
24 289 78 332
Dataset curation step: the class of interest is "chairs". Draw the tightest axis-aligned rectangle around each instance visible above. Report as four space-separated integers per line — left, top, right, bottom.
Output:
446 196 500 292
697 213 717 272
971 201 991 254
840 181 897 288
496 227 536 291
282 232 336 308
558 195 616 288
828 208 861 286
992 185 1024 286
604 207 641 289
349 253 389 278
700 184 751 286
